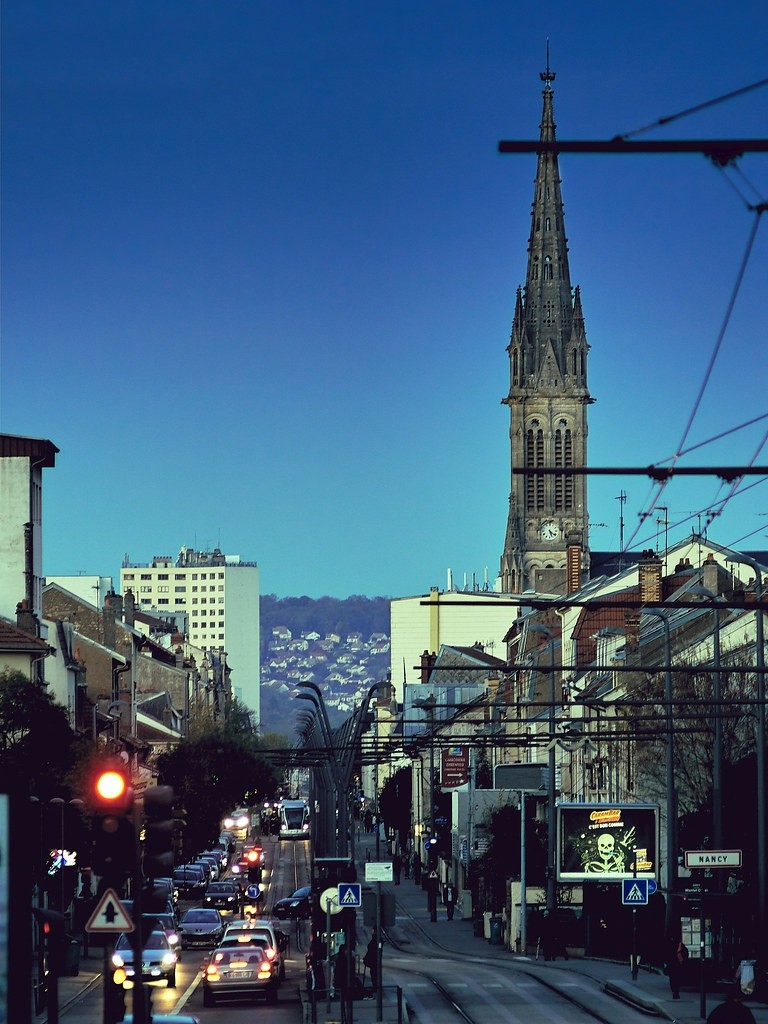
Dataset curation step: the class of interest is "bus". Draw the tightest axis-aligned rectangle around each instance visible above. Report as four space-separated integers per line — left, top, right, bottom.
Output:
278 799 311 838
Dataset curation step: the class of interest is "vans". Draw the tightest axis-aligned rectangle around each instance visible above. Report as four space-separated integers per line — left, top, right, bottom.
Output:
224 873 249 894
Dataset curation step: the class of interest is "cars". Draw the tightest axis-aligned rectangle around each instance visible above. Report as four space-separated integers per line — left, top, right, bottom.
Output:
233 858 252 875
140 904 183 961
219 920 278 966
200 947 280 1006
272 885 313 922
113 929 176 987
208 935 278 977
203 881 239 915
140 829 236 903
238 845 266 869
178 908 226 948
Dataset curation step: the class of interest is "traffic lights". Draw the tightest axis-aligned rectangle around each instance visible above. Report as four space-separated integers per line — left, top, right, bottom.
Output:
93 769 126 877
39 919 59 937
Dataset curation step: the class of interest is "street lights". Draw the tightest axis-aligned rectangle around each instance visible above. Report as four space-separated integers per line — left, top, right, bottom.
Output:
526 622 560 959
639 607 681 1001
383 744 423 888
414 698 437 921
687 584 723 988
726 553 768 1000
295 681 394 1023
49 797 85 937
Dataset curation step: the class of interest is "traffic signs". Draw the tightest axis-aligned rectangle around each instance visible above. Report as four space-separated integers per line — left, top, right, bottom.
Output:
622 878 649 906
339 883 363 907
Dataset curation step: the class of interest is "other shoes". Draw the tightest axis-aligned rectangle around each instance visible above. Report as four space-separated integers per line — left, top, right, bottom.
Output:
394 882 400 886
447 918 453 921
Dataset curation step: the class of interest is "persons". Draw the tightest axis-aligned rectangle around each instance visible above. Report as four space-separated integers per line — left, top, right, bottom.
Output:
261 820 269 837
362 809 372 833
421 650 437 660
362 924 383 1001
392 855 402 887
534 910 557 961
402 853 411 880
331 944 356 988
443 885 462 921
302 952 319 1003
386 843 392 856
412 851 422 886
353 805 359 821
